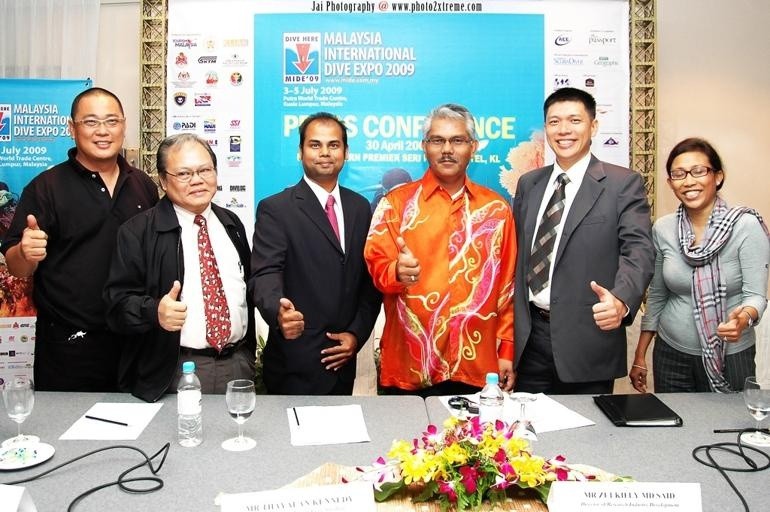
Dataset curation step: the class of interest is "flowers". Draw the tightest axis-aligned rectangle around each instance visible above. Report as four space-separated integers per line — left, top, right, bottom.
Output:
351 417 627 507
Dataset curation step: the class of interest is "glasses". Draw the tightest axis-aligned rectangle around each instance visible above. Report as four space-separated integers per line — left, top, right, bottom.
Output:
74 117 126 128
425 137 472 144
165 168 218 183
669 166 717 180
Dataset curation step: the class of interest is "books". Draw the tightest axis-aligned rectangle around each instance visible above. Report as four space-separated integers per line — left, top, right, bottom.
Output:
596 392 684 431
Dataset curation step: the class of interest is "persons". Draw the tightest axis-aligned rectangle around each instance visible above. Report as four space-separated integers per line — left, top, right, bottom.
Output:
361 104 517 398
249 112 383 396
100 132 258 403
0 87 164 391
513 89 656 396
627 137 768 393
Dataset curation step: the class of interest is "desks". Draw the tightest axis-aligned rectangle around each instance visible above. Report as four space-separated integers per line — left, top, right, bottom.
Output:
0 394 426 510
427 393 770 512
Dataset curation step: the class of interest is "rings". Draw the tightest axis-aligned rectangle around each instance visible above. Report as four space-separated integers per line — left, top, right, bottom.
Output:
721 334 729 346
409 273 415 281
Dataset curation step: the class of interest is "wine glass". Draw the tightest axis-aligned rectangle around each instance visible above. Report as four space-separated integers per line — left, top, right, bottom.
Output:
221 379 257 452
741 376 769 447
2 378 40 446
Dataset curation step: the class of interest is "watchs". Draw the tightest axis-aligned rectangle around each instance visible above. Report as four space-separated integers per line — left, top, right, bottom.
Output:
743 311 756 335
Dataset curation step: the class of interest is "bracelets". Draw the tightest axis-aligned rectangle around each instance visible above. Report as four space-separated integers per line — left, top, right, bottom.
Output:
632 363 650 372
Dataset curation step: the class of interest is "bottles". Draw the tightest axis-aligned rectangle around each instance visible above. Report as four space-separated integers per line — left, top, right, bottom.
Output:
478 371 504 422
177 362 203 448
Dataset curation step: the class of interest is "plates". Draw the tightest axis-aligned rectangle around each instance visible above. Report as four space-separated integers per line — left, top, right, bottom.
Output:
0 443 55 474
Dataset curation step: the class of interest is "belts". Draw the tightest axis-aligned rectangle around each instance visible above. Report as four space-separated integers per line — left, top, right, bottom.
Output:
530 302 550 322
180 339 248 358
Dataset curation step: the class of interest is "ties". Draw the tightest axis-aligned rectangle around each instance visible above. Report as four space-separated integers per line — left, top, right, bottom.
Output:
194 215 231 353
325 195 340 242
527 173 570 296
